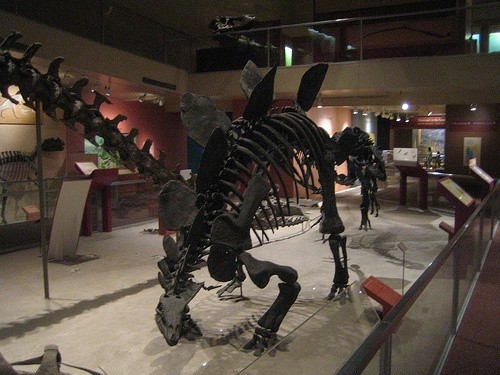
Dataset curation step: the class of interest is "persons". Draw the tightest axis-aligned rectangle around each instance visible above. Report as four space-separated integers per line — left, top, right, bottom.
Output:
434 149 441 170
425 146 433 171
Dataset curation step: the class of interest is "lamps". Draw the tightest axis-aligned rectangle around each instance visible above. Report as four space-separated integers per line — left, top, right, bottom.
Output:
317 97 324 110
104 85 112 96
89 79 101 93
352 106 410 123
138 93 147 103
153 95 165 107
